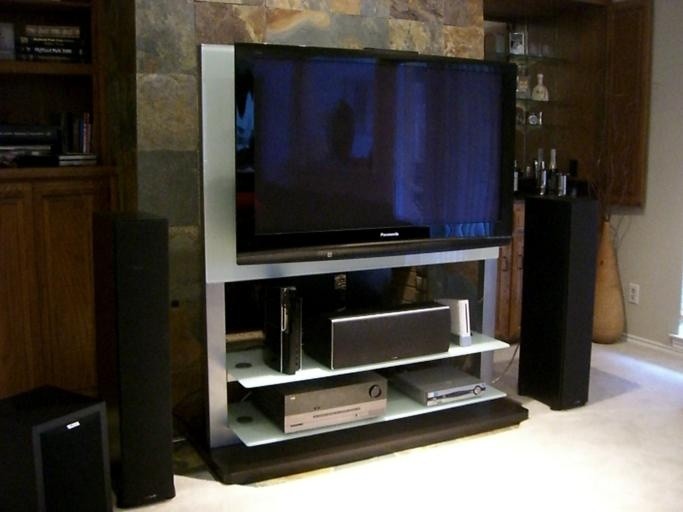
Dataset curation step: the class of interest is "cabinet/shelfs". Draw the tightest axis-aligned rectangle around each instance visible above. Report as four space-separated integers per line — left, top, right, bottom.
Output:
483 1 654 344
1 1 119 395
226 331 510 449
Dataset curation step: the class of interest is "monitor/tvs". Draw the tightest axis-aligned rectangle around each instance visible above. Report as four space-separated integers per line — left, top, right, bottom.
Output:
234 41 517 265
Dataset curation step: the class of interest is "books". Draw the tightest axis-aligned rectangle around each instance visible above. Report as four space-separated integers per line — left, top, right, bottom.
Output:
22 25 83 39
18 45 82 54
0 22 16 61
20 36 79 47
0 109 99 166
19 52 83 65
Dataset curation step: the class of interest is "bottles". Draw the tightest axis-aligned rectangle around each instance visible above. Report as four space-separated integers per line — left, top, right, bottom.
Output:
531 74 549 101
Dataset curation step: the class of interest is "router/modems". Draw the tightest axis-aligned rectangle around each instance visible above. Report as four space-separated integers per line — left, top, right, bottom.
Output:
436 298 472 346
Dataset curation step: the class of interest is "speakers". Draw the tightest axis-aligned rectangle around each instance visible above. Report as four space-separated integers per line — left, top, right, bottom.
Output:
263 285 303 374
90 207 176 508
0 383 114 511
307 295 451 372
517 196 600 411
